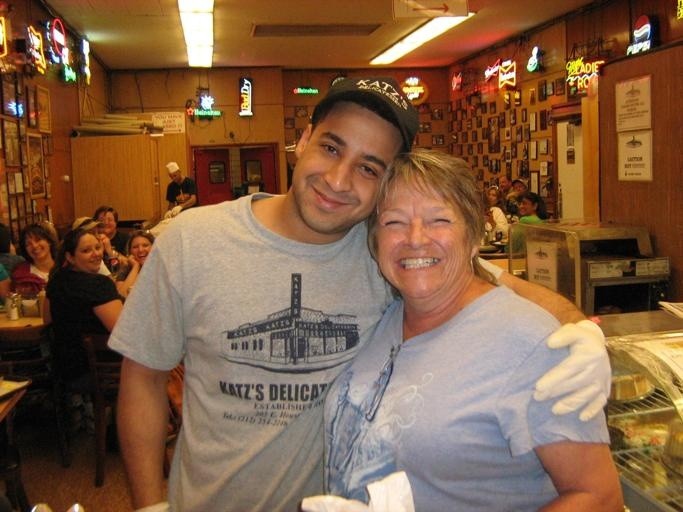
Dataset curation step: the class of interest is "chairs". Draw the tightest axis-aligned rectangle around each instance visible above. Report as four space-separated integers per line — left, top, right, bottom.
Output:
0 321 70 467
82 333 174 488
1 373 39 511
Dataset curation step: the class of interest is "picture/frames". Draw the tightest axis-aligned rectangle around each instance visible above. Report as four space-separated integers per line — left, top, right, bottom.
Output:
26 132 46 199
1 72 26 120
244 159 262 181
25 86 37 129
416 77 565 194
208 160 225 184
35 85 51 135
0 118 22 166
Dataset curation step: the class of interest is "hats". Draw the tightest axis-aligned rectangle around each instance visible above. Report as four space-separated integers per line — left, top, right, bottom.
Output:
512 179 528 189
72 216 104 234
166 162 179 173
313 76 419 150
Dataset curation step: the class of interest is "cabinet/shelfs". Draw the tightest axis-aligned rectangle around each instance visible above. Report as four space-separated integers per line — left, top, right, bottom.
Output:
592 309 683 512
68 135 161 223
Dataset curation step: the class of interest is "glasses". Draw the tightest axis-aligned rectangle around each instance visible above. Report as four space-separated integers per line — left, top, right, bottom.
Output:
365 343 401 421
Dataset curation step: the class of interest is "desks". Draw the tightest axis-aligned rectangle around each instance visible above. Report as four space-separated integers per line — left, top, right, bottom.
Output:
477 245 526 279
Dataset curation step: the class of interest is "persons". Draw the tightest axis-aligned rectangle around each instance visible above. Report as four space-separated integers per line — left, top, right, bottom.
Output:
164 161 199 218
485 184 508 233
105 75 612 512
503 192 545 253
506 177 529 215
0 206 154 372
301 147 623 512
498 174 513 195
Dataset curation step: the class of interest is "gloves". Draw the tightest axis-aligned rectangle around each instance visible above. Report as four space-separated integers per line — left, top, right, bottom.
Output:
532 319 613 422
164 206 182 219
